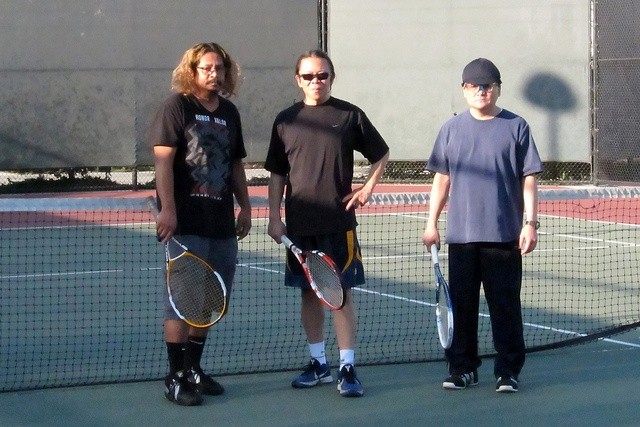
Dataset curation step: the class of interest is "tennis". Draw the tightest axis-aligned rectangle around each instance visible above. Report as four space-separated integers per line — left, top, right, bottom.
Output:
235 223 243 232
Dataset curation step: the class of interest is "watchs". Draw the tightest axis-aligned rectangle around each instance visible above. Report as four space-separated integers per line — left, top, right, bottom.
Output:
525 220 541 230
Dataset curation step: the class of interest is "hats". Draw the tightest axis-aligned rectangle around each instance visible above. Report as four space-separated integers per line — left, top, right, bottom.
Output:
462 57 499 84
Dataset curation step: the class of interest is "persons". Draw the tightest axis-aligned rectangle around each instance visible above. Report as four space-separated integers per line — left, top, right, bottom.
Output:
150 41 252 405
265 47 389 395
419 56 544 393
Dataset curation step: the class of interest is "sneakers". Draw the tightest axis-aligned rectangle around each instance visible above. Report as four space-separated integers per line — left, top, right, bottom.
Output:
495 376 520 392
291 358 334 388
164 370 202 406
336 363 364 397
189 369 224 396
442 368 479 389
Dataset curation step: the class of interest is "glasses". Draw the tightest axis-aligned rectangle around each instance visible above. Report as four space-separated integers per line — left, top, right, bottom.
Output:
462 83 500 90
197 66 226 73
298 72 329 80
